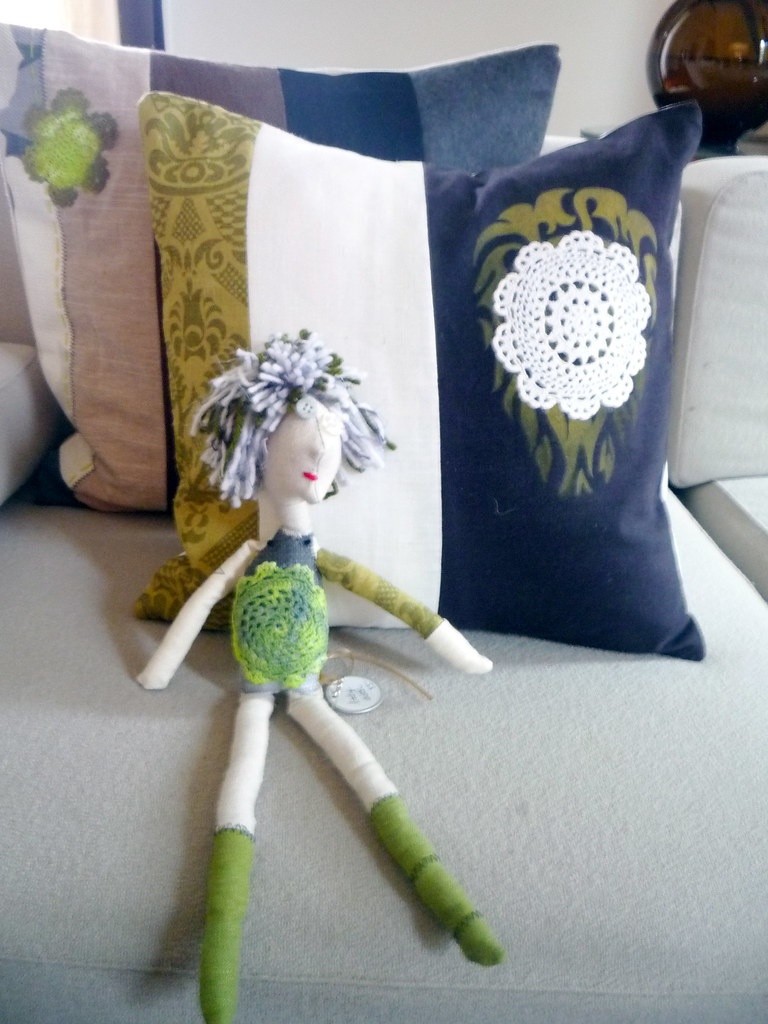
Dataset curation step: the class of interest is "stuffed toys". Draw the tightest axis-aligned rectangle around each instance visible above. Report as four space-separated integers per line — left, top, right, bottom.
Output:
137 330 501 1024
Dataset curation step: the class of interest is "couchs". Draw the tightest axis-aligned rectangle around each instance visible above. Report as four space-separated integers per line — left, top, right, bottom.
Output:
0 135 768 1024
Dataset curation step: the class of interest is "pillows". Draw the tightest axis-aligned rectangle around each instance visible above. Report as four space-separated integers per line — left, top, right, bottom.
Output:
137 90 704 675
0 20 563 512
